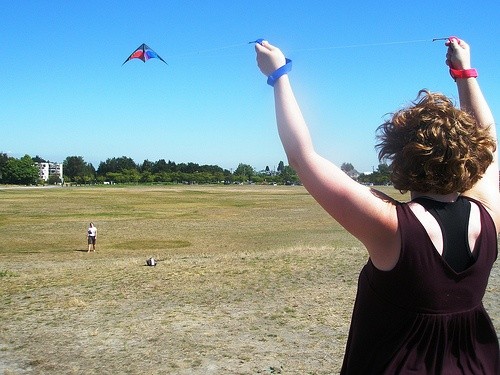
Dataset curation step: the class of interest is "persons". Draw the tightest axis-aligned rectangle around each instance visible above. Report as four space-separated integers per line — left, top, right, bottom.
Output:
256 38 500 375
86 222 97 252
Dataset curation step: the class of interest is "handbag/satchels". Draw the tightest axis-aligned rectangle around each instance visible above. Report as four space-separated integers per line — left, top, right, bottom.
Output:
147 257 156 265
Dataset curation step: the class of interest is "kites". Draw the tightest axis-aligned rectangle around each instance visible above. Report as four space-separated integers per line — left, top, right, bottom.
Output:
122 43 169 67
